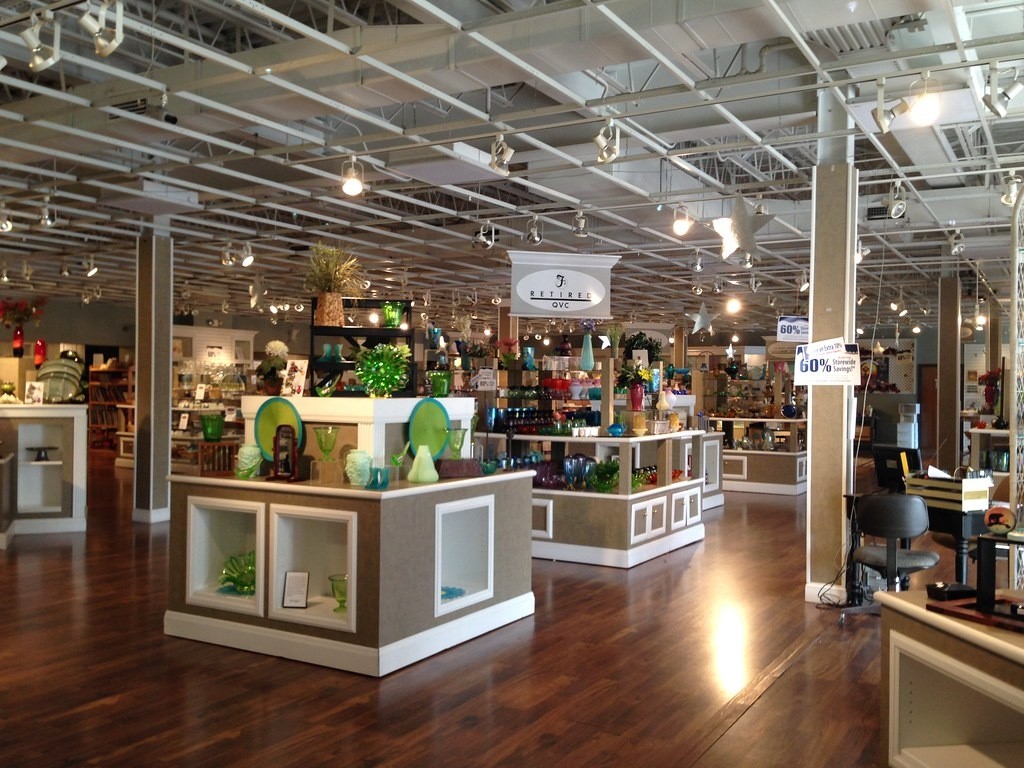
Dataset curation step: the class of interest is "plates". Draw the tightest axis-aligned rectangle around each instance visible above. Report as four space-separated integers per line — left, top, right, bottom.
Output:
648 469 683 484
408 397 450 460
254 397 303 462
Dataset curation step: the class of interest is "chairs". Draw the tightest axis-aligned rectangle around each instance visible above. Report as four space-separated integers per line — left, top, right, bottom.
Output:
837 493 940 627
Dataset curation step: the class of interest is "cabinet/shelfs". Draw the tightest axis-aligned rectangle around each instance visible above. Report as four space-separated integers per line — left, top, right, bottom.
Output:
873 589 1024 768
307 296 417 398
969 426 1011 507
0 357 808 677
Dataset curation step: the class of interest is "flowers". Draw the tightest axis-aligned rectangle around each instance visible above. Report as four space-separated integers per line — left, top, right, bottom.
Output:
255 340 289 378
578 318 601 334
0 297 48 329
618 365 653 388
496 338 520 355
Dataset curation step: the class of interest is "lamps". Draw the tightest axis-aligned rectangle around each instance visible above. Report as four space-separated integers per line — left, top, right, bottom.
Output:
672 171 1023 343
871 60 1024 134
0 0 123 72
488 134 514 177
358 265 501 336
175 240 304 325
0 195 101 305
594 118 620 165
472 211 589 250
341 155 364 196
524 318 575 346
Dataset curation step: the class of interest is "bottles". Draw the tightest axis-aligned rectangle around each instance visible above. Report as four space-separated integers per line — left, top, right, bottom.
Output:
551 418 586 435
344 448 372 486
236 443 262 476
1 381 15 391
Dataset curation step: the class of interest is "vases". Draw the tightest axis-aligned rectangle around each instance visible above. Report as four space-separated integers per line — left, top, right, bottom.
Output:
13 327 24 358
263 378 283 396
314 293 345 328
35 338 47 369
631 383 643 411
580 334 594 371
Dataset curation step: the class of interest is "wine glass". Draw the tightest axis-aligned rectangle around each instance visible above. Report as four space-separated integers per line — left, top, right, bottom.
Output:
313 425 339 462
328 573 348 613
442 428 467 459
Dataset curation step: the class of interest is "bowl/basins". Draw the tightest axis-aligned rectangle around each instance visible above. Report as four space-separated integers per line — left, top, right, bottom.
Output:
632 428 648 436
631 472 647 491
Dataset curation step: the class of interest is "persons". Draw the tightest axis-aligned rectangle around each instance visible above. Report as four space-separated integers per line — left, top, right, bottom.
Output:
27 384 36 402
285 362 302 394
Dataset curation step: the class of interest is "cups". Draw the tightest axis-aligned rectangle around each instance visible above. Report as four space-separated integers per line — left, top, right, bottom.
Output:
428 328 442 348
430 371 450 397
380 301 405 328
563 452 596 492
482 461 497 474
199 414 224 441
367 467 389 489
